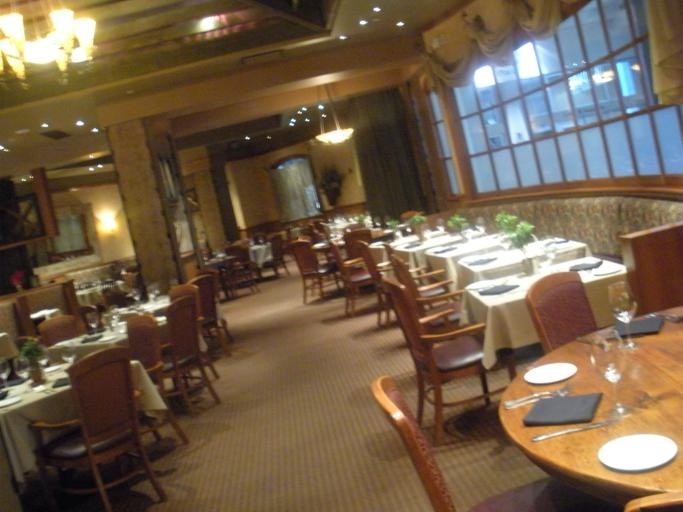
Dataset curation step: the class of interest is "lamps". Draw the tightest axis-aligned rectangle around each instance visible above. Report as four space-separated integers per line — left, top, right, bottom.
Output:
0 0 97 79
315 83 354 145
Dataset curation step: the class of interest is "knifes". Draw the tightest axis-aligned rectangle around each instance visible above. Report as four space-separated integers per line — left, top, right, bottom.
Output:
531 421 615 442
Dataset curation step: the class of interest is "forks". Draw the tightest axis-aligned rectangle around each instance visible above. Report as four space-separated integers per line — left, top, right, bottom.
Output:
503 382 573 410
645 311 683 323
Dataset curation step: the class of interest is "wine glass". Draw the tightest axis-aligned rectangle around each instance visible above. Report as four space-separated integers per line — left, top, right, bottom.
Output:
607 280 641 352
462 216 487 245
84 282 160 341
589 329 634 421
0 342 78 413
323 210 392 246
499 238 512 258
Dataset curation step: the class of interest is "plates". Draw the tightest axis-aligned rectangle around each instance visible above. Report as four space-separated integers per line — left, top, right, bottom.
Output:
465 279 505 290
597 433 679 473
523 361 578 384
592 266 622 277
